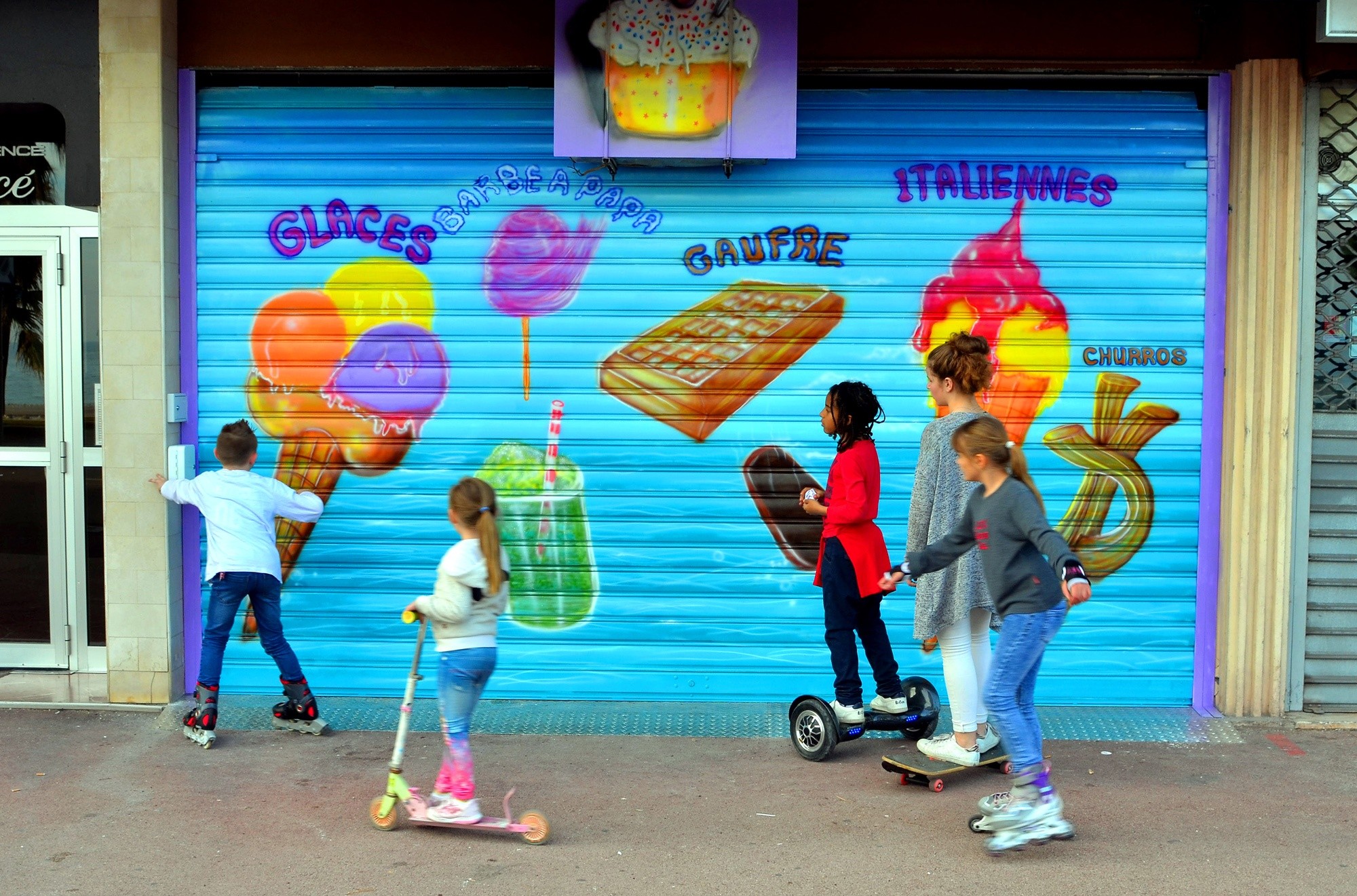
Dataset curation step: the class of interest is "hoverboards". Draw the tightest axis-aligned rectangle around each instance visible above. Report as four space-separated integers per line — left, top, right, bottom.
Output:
367 608 552 846
788 676 941 763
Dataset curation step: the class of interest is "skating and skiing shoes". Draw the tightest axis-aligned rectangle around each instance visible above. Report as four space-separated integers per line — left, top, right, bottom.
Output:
968 760 1075 855
181 682 220 749
271 674 329 736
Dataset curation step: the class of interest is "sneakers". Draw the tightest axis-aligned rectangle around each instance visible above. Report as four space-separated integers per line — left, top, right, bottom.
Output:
430 791 452 806
424 795 483 824
828 700 865 723
975 721 1001 753
870 692 908 714
917 733 980 767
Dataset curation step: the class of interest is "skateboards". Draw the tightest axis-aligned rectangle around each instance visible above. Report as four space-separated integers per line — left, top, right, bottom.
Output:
882 745 1013 792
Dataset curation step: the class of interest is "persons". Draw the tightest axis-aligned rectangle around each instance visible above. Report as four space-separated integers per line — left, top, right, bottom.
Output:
400 478 509 824
151 421 321 730
798 381 910 721
905 332 1019 767
878 416 1091 835
